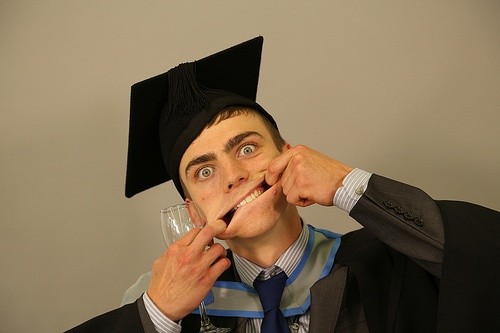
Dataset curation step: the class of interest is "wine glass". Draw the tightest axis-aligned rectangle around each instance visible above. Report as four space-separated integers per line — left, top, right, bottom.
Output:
160 202 232 333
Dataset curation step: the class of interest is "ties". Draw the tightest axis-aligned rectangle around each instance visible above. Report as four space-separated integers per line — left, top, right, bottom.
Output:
253 271 291 333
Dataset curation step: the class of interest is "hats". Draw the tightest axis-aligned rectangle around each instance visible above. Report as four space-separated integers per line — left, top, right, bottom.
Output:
125 36 265 200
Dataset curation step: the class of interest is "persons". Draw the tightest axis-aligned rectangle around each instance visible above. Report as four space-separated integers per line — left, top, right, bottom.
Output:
64 37 500 333
